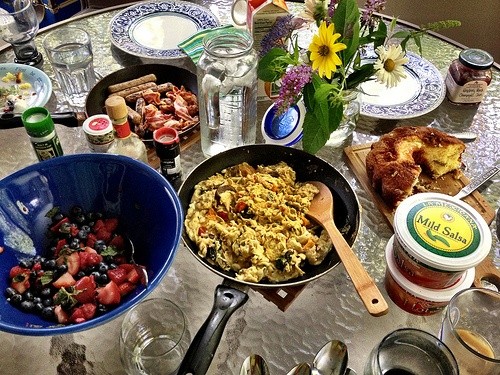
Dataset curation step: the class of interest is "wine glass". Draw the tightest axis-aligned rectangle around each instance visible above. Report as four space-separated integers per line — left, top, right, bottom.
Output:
0 0 43 66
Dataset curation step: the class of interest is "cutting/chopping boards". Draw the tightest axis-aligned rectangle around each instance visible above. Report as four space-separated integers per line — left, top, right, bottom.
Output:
343 142 500 298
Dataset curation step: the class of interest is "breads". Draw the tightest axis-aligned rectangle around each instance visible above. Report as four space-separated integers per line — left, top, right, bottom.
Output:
365 125 466 206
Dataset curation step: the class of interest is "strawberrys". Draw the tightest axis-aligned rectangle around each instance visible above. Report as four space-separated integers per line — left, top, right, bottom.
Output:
9 217 146 324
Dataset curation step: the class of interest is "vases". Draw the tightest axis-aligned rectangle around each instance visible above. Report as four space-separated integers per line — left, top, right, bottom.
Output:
322 87 360 148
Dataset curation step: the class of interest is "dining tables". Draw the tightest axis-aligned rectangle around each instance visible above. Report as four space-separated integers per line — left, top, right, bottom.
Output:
0 0 500 375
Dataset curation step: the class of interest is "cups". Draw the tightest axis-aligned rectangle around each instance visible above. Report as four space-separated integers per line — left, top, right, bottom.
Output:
438 287 500 375
363 327 460 375
118 297 192 375
42 27 96 109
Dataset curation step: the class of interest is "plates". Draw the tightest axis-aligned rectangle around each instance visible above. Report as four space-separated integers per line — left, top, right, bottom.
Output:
106 0 220 59
338 45 447 120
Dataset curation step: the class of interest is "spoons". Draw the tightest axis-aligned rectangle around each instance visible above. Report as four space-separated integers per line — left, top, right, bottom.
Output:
312 339 349 375
286 362 311 375
239 353 271 375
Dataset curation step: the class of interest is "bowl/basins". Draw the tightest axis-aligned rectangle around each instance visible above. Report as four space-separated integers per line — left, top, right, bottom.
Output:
0 154 182 336
0 63 53 118
392 192 493 289
384 233 476 317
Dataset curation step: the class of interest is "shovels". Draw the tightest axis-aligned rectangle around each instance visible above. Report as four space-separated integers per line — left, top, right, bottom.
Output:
302 181 390 318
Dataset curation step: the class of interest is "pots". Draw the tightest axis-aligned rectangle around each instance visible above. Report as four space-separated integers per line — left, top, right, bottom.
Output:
0 63 207 147
176 143 360 375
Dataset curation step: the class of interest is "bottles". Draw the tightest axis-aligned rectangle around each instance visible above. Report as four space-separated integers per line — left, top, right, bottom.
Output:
197 27 259 158
105 95 148 165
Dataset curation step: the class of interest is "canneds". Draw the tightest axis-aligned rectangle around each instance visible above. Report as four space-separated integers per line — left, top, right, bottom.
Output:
445 48 494 106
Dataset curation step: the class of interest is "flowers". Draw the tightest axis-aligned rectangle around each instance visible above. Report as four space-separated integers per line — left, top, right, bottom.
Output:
258 0 462 154
